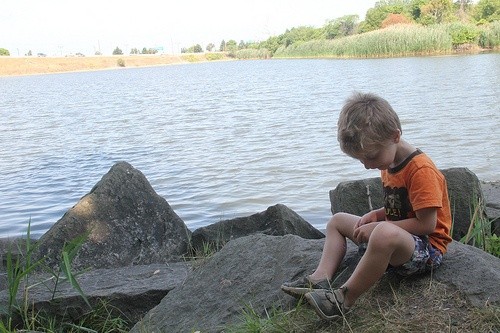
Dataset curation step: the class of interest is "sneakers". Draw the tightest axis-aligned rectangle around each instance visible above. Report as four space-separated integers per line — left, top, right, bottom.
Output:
281 274 334 300
304 285 352 321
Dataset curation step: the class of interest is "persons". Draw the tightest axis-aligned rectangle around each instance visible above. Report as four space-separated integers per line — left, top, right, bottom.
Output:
279 90 453 320
